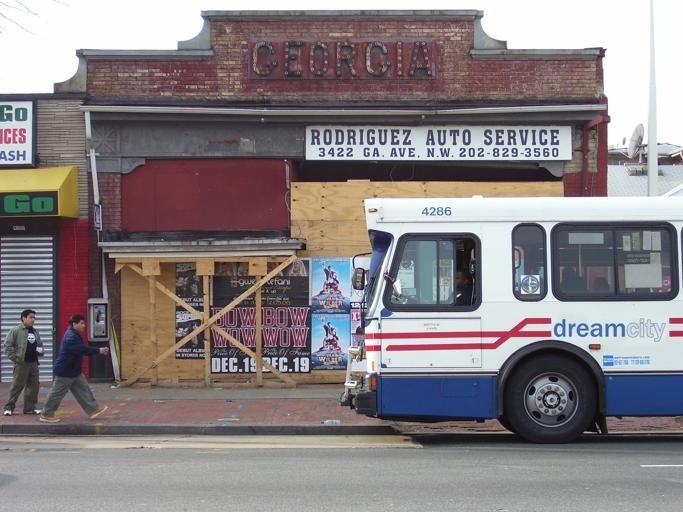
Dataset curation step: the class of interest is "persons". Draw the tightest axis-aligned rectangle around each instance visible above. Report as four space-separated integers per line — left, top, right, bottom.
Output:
322 321 341 364
289 261 306 277
39 313 109 423
2 310 44 417
444 270 472 305
589 276 609 291
318 263 342 299
561 267 587 292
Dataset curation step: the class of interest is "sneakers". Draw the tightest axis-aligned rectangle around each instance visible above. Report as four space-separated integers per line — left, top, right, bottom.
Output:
88 405 109 419
39 413 61 423
23 409 43 415
3 409 12 417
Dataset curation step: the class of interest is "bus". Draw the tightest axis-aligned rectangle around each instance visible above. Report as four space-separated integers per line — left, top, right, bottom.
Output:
338 189 682 450
